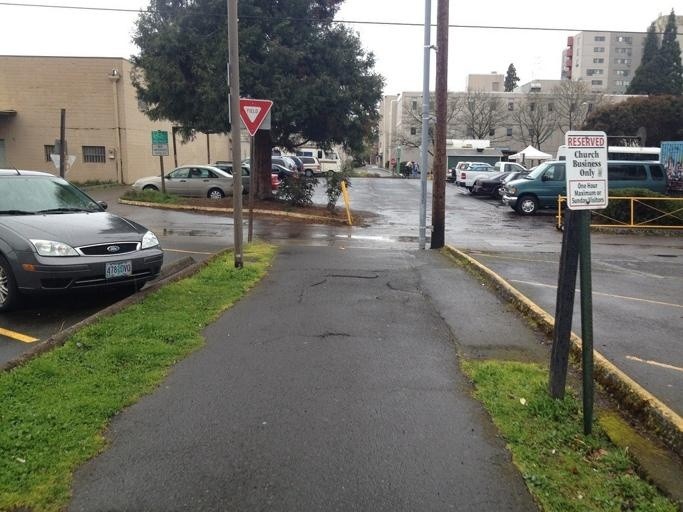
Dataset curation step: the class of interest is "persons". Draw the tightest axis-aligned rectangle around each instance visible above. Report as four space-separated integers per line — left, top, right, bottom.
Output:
404 160 413 178
411 160 418 178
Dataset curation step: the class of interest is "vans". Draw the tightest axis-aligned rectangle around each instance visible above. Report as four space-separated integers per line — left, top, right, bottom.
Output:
496 159 669 218
553 144 662 163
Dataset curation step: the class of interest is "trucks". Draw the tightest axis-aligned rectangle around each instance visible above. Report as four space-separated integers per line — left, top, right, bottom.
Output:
294 145 342 178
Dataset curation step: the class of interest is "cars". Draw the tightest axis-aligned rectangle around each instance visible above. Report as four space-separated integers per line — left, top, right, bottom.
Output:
271 164 296 182
447 161 528 198
271 174 282 191
0 157 165 311
131 163 245 202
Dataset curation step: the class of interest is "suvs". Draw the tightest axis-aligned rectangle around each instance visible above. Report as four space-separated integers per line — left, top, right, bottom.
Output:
296 156 323 177
290 156 305 177
207 159 249 193
240 156 298 178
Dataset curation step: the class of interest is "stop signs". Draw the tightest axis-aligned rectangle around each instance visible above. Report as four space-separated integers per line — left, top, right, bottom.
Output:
389 157 397 167
